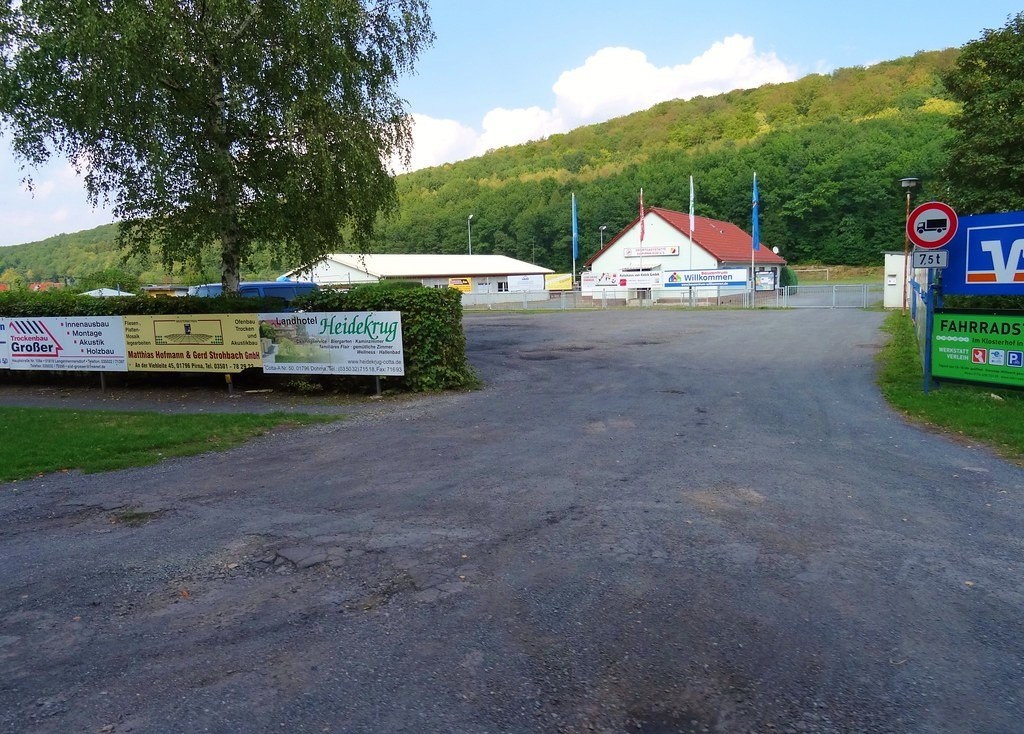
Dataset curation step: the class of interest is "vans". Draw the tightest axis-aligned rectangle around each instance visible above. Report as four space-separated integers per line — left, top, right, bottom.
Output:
188 282 319 312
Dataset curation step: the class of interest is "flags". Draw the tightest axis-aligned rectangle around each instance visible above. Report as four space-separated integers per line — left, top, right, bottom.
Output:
689 177 694 231
752 176 760 251
573 197 578 258
640 189 644 241
